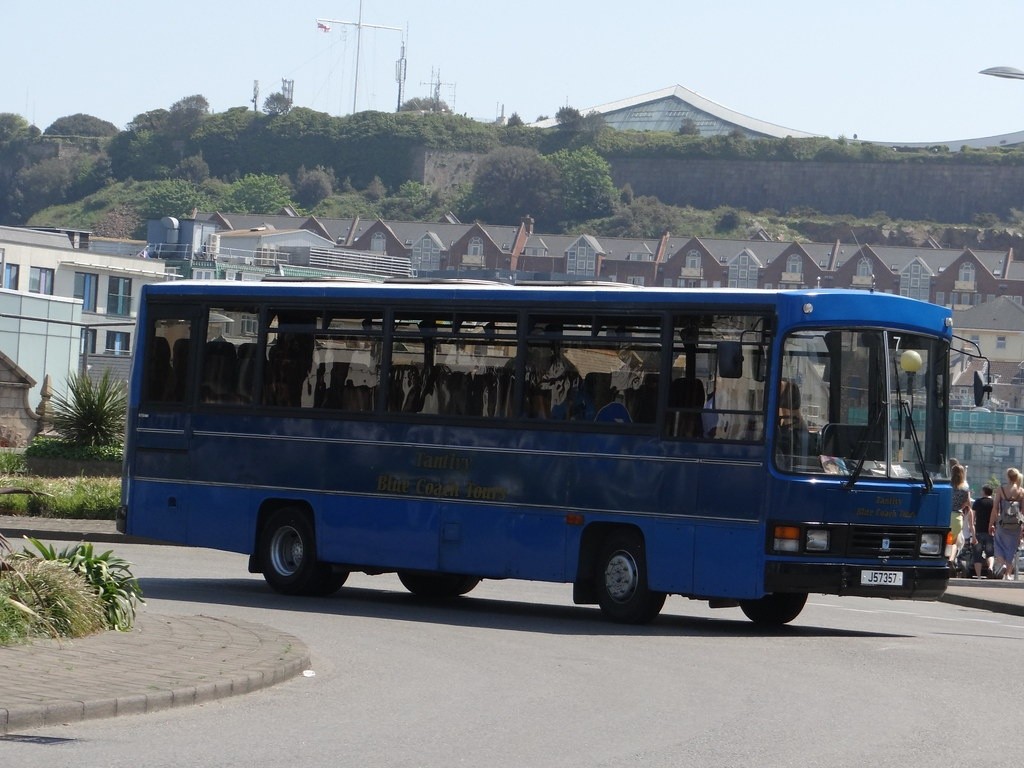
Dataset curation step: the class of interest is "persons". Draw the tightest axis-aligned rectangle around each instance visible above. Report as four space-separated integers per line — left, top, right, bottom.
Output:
949 458 1024 580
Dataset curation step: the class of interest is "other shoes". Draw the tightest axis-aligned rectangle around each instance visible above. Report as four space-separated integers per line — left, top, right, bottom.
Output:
996 565 1007 577
987 568 993 579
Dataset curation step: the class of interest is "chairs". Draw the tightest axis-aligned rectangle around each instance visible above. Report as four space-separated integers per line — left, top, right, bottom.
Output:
154 337 705 437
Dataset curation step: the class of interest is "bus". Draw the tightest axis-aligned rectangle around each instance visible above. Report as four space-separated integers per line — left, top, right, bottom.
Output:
116 281 993 624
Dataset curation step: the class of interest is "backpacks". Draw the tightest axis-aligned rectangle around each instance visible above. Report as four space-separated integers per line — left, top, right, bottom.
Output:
1001 487 1024 529
